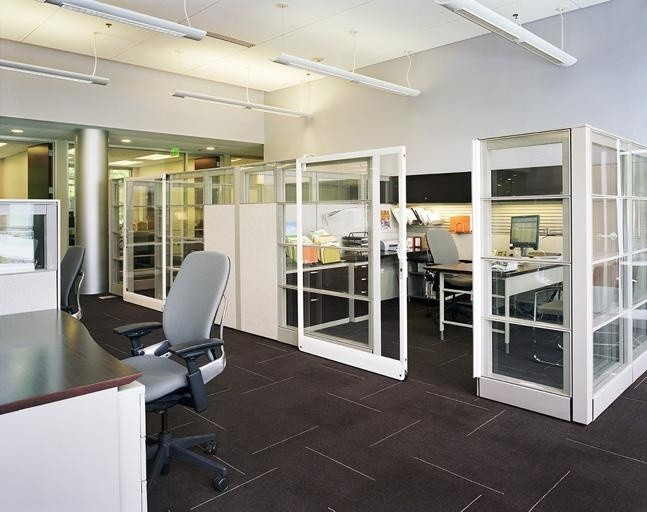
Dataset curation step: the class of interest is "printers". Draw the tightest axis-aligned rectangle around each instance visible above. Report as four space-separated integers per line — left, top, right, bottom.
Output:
380 239 398 251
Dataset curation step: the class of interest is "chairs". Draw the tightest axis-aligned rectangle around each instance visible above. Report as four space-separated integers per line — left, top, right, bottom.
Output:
112 251 232 491
60 245 86 321
532 282 564 368
425 229 472 324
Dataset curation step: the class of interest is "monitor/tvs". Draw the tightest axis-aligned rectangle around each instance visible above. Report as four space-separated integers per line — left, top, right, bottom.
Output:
510 215 540 257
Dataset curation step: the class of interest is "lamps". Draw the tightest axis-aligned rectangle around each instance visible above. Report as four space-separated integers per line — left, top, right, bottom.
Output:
438 0 577 67
269 50 421 101
168 67 311 117
1 58 111 87
43 0 208 43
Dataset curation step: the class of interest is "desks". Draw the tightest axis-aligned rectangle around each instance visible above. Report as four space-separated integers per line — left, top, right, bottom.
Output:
423 251 564 354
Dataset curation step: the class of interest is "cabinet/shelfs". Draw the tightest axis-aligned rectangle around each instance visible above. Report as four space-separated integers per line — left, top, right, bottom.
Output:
407 257 433 305
286 261 369 332
0 308 148 512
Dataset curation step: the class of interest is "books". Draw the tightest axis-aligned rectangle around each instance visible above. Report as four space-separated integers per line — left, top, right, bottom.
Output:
286 229 341 264
